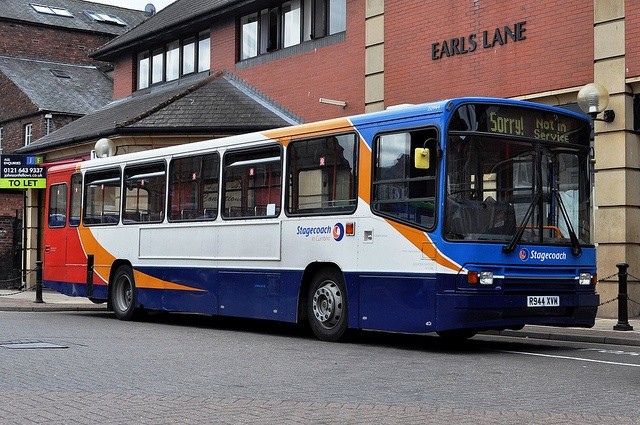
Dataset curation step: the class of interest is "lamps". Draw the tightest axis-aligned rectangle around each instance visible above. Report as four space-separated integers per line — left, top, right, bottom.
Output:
95 138 117 158
577 83 615 123
318 98 346 107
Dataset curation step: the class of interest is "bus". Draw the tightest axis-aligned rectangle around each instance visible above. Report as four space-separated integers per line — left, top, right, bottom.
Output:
42 97 600 343
